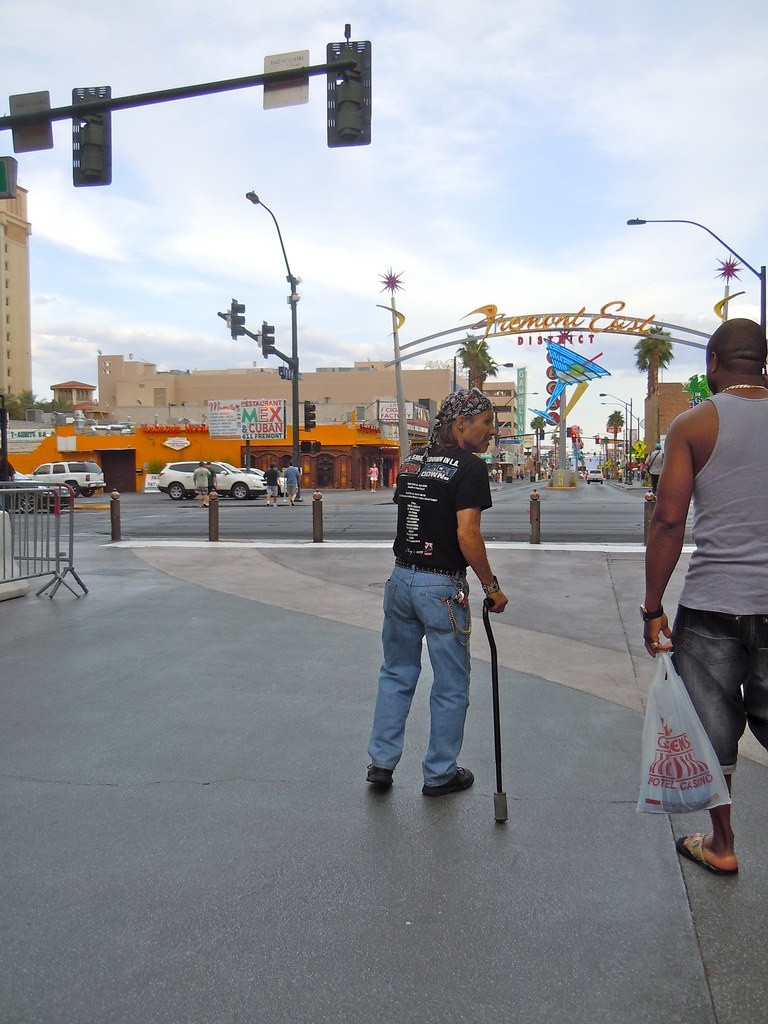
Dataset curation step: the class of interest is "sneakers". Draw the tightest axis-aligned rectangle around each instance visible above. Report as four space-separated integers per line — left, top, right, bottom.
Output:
366 764 393 783
422 767 474 796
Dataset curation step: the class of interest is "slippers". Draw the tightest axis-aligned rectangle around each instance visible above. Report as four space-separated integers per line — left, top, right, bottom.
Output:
677 832 738 876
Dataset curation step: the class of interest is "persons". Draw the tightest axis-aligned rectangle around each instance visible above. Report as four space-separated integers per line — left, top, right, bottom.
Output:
646 443 664 494
639 461 648 482
617 468 623 483
516 470 525 480
205 462 215 498
0 449 16 513
284 460 301 506
627 469 635 480
193 461 211 508
263 462 280 508
365 388 510 796
367 462 379 494
638 319 768 876
491 467 503 484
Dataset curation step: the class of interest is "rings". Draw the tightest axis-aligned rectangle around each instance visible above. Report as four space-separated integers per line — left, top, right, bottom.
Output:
651 641 658 649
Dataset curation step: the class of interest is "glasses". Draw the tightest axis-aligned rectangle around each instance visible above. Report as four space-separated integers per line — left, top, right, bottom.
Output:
460 387 483 416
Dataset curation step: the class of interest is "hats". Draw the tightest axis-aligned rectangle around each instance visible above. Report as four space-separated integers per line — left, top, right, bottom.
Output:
655 444 662 449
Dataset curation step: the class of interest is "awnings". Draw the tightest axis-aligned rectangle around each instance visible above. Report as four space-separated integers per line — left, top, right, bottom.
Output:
408 436 429 445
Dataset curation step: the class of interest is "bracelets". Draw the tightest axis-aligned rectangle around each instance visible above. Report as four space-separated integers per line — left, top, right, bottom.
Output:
481 575 499 595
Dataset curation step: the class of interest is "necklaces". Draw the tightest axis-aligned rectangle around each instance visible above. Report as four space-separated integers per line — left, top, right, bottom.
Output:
722 383 767 395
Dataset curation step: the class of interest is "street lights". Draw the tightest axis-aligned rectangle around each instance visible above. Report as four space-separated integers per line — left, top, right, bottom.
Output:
599 393 633 485
624 218 766 333
494 392 539 483
600 402 642 482
245 189 304 502
468 363 514 390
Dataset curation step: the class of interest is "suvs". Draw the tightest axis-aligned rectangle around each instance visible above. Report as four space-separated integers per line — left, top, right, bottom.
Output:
158 461 267 500
25 460 107 498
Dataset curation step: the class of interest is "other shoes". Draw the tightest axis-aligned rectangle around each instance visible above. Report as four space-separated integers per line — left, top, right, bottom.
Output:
203 502 209 507
290 500 295 506
371 490 376 492
274 504 278 508
267 501 270 507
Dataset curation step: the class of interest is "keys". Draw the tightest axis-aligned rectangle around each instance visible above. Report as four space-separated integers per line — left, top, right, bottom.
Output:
454 589 468 609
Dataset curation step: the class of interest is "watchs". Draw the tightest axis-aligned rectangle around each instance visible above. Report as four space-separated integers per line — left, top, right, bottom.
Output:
639 604 663 621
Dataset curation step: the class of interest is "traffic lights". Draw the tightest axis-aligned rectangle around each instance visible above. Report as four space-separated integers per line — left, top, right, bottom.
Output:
262 320 275 359
304 400 316 432
230 300 245 341
596 435 600 444
572 433 577 442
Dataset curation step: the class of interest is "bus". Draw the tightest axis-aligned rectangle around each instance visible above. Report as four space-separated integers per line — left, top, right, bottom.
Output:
578 466 587 479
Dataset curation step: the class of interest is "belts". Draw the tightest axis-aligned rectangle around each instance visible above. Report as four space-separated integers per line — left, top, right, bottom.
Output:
395 558 467 578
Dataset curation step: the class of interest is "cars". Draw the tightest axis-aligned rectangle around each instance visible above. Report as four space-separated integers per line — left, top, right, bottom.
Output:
237 468 290 497
4 470 71 515
587 469 604 484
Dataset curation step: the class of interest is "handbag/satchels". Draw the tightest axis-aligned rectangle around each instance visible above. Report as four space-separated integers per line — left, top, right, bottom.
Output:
635 644 732 815
647 467 650 473
